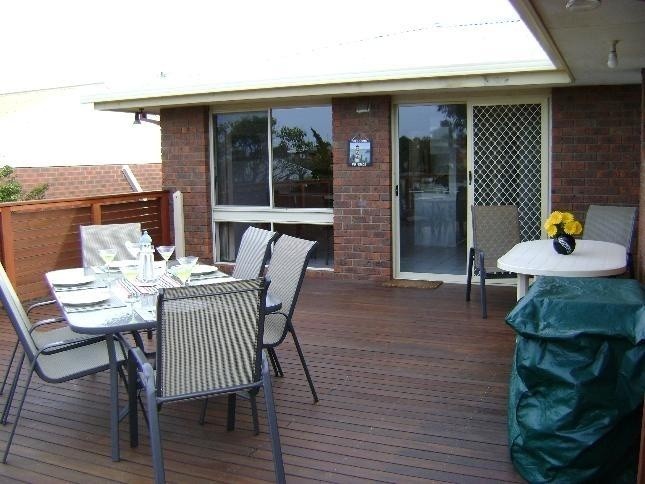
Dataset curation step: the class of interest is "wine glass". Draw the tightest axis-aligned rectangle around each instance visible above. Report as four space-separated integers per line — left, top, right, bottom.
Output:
100 248 118 278
127 242 141 271
177 255 198 285
175 268 193 287
156 246 175 274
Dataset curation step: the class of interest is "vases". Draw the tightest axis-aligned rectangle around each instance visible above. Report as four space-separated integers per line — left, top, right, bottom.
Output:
553 233 575 255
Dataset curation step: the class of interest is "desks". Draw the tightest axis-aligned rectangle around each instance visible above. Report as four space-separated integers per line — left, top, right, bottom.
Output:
497 240 626 302
235 179 332 256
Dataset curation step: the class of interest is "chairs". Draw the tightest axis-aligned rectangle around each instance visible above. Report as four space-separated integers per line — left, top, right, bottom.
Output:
456 185 466 240
466 205 522 320
583 204 639 278
295 178 330 265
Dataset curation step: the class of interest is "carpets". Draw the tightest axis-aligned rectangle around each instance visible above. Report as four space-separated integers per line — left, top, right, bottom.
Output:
382 279 443 289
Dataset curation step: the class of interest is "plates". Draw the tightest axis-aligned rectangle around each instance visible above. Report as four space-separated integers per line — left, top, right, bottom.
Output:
60 291 113 305
104 260 140 269
52 273 95 286
172 265 218 275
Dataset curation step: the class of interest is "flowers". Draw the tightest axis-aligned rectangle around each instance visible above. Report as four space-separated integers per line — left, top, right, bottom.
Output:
541 210 583 238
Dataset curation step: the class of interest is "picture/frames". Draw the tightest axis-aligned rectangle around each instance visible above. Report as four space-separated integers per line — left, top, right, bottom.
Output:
346 139 373 166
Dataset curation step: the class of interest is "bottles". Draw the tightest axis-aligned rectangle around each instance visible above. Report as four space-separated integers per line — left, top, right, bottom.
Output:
140 231 156 283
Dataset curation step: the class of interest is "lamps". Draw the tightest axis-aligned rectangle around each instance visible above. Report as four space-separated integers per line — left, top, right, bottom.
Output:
134 108 147 124
564 0 601 10
607 40 618 69
